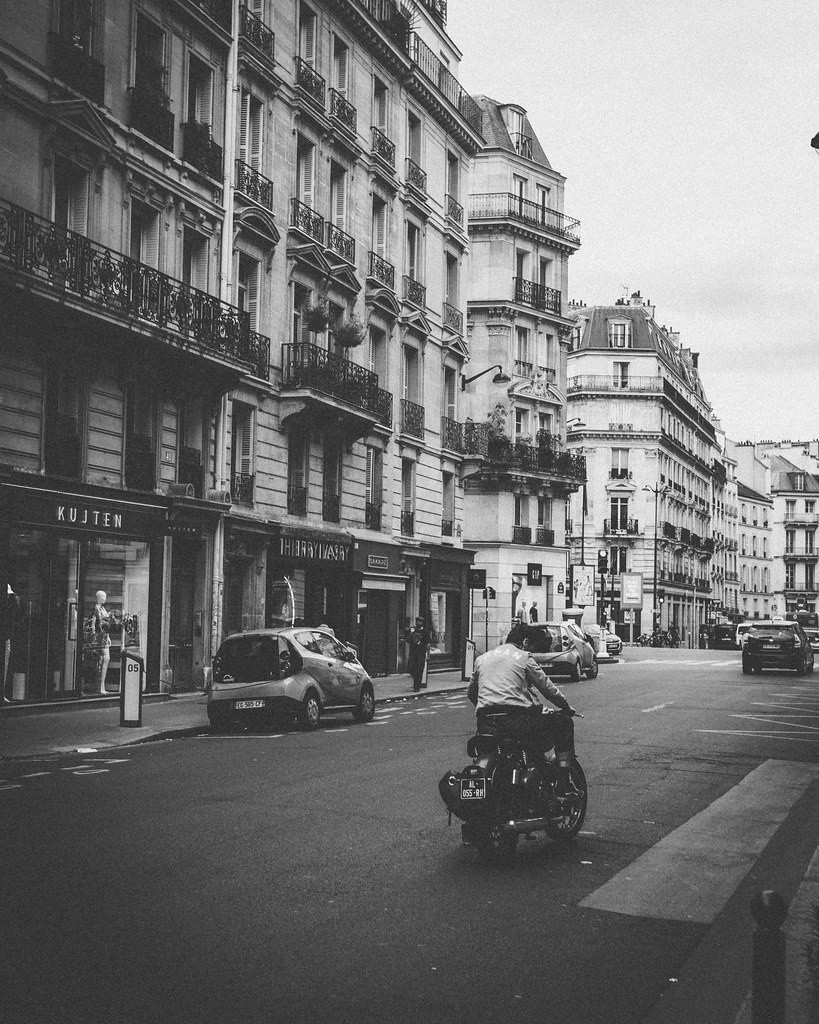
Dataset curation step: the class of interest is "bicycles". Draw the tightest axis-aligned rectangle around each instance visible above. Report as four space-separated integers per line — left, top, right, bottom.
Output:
636 622 682 648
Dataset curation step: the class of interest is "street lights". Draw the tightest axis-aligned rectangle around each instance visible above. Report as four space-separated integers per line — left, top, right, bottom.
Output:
642 481 672 631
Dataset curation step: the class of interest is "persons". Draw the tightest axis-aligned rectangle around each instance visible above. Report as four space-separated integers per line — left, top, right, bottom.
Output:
667 621 679 648
91 590 114 694
467 625 584 802
399 616 431 692
317 614 336 637
652 627 660 646
3 584 20 701
518 601 528 624
530 601 539 623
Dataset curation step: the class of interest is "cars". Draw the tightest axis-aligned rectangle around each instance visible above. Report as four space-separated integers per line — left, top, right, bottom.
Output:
527 621 599 682
207 627 375 733
699 623 753 650
742 620 814 675
803 630 819 654
583 623 623 655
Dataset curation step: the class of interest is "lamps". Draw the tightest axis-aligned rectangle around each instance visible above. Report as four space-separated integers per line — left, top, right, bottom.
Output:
462 365 511 392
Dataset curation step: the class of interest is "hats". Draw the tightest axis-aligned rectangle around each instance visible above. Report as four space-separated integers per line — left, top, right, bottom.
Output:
416 616 424 621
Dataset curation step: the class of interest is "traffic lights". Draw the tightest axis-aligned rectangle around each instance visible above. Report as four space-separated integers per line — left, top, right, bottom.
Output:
658 588 665 603
598 550 609 574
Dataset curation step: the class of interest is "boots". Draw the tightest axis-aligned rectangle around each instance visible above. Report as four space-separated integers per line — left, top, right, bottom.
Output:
557 756 585 801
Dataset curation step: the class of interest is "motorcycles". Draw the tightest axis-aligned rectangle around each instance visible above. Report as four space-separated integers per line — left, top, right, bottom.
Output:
438 705 588 860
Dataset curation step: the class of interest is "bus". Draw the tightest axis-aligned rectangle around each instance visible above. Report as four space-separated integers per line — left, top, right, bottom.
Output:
786 613 819 649
